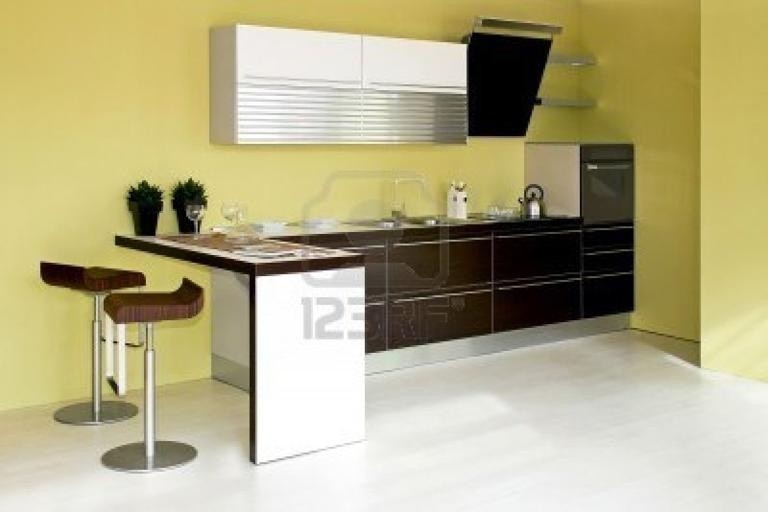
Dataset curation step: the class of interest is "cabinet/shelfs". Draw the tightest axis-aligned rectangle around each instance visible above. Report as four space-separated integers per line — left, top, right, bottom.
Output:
387 224 494 349
580 221 634 318
275 229 387 353
495 219 584 333
209 23 469 145
536 55 597 107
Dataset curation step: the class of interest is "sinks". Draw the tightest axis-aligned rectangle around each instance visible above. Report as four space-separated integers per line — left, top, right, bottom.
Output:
403 218 442 225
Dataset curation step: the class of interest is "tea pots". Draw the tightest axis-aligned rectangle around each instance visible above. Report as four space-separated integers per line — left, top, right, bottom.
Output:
518 184 546 221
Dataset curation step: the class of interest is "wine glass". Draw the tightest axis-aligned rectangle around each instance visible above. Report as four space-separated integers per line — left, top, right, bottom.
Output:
221 200 245 238
186 203 205 240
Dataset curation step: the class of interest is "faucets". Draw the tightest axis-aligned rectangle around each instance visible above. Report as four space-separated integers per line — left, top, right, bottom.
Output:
394 177 426 210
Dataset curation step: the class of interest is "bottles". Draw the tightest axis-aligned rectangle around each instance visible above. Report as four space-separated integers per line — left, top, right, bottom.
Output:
446 178 467 219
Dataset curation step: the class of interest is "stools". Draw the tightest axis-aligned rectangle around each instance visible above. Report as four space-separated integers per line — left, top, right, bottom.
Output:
101 277 205 472
39 261 145 425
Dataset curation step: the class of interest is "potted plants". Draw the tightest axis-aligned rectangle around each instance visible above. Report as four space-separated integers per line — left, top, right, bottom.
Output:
171 177 208 233
127 180 164 236
